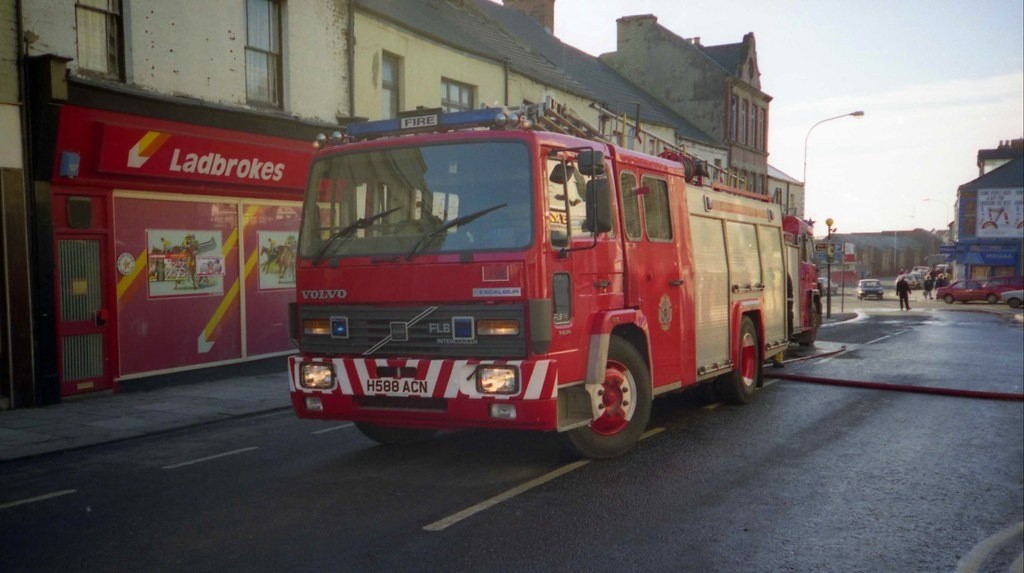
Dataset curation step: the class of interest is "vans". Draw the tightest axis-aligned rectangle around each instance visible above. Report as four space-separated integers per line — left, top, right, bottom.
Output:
983 275 1024 290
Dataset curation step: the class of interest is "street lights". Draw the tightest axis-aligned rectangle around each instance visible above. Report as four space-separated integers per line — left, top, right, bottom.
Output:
894 216 915 277
826 219 837 319
923 198 949 242
803 111 864 219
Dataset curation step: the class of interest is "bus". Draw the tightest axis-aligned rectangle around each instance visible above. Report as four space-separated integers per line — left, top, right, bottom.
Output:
924 254 949 270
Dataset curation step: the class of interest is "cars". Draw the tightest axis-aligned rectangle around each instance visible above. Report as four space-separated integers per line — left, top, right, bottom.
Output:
937 280 1024 308
895 263 950 289
817 277 838 297
856 279 885 300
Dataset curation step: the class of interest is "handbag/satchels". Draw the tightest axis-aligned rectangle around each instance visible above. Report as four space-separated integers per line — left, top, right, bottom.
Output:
923 289 928 296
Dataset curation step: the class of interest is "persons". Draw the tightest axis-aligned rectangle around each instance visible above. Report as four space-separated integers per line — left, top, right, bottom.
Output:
924 268 949 299
895 276 912 311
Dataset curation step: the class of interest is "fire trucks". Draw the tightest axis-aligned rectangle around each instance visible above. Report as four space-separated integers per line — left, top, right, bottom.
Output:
286 96 822 460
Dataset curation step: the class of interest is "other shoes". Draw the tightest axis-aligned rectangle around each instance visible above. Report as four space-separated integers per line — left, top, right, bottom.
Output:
901 308 903 311
907 308 911 311
930 298 934 300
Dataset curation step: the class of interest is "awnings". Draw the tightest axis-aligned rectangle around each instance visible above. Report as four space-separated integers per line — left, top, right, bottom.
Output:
956 250 1019 265
